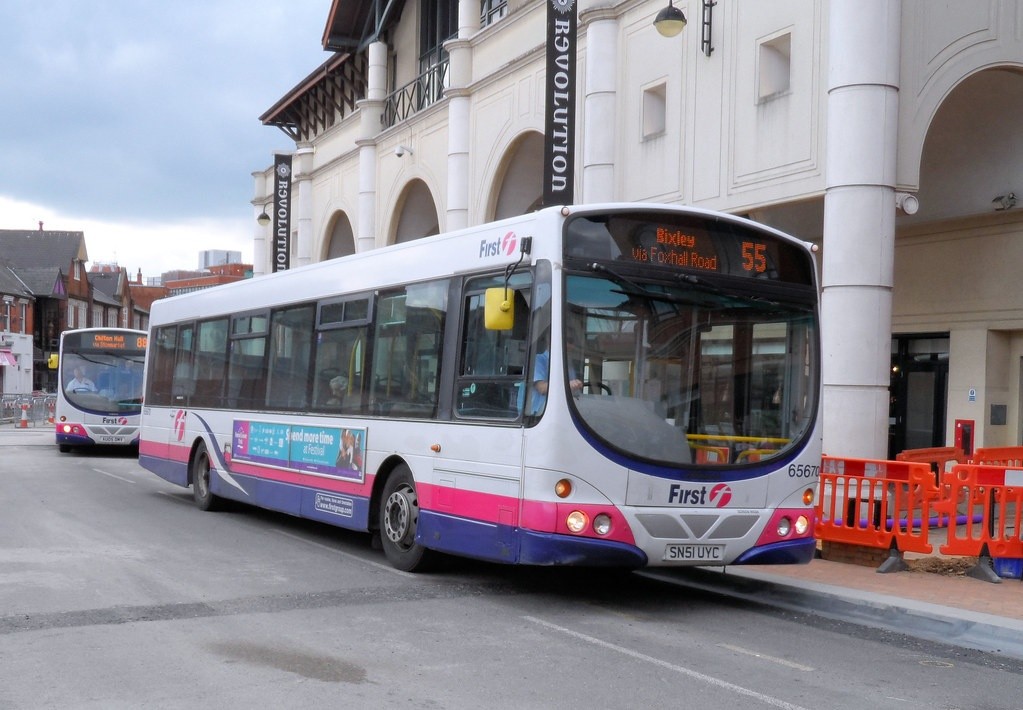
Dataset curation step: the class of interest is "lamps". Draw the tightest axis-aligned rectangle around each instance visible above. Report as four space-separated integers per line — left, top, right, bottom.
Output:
653 0 718 59
257 201 274 226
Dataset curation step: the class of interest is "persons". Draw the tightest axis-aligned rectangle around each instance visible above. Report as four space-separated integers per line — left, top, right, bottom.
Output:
516 330 584 415
336 429 362 471
66 367 95 392
328 377 348 406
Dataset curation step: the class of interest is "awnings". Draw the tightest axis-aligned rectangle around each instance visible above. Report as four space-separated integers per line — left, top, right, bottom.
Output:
0 349 20 366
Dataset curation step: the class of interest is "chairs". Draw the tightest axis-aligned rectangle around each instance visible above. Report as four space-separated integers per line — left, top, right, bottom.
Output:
165 364 401 420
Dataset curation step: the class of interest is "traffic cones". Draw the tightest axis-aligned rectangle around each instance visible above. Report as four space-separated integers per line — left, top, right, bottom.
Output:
21 404 27 428
49 402 55 424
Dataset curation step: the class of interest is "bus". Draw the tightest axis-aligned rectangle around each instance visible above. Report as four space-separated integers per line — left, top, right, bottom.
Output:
140 202 825 573
48 327 151 453
